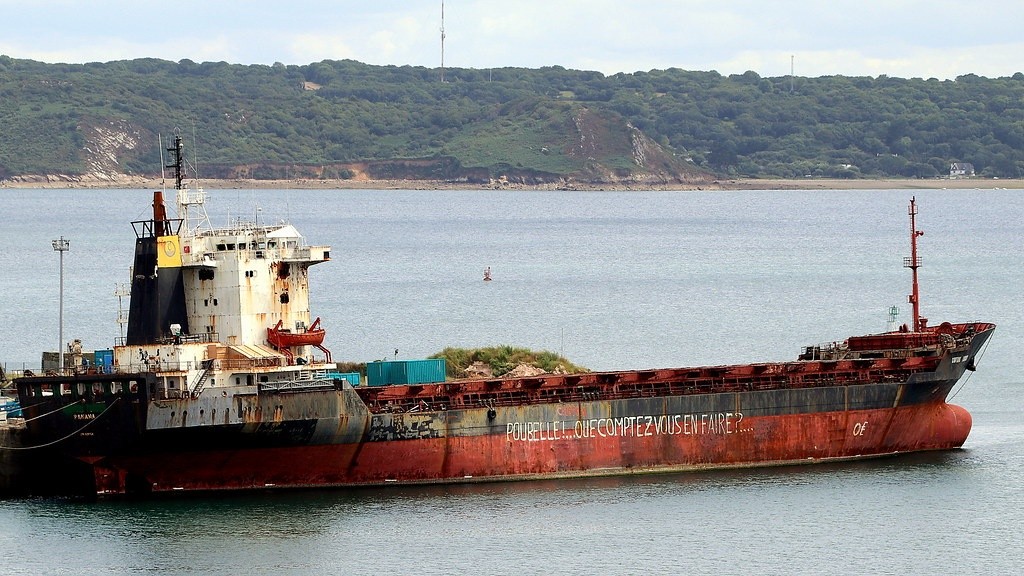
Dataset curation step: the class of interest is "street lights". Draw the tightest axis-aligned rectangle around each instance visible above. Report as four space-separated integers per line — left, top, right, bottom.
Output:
47 235 70 376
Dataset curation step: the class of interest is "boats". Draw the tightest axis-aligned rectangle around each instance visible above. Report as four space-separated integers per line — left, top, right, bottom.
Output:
266 317 325 348
12 128 999 496
